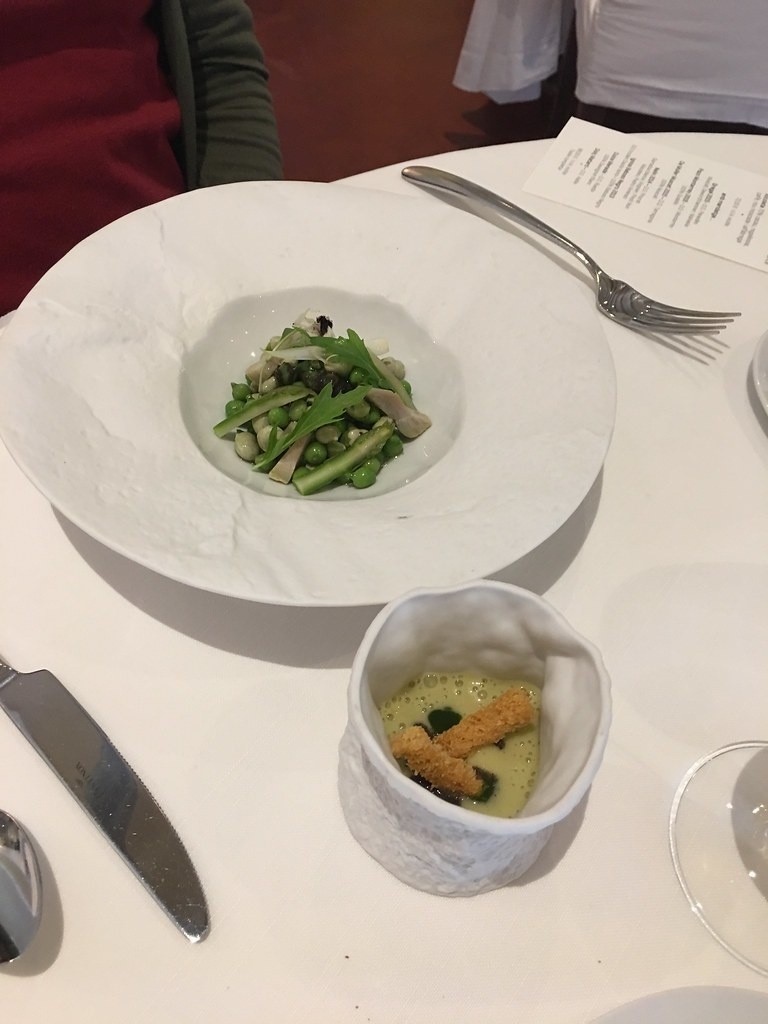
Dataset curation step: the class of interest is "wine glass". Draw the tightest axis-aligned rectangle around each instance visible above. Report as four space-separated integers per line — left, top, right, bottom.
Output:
666 736 768 983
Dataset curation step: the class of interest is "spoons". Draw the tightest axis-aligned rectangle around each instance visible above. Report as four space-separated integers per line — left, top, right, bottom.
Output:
0 808 45 962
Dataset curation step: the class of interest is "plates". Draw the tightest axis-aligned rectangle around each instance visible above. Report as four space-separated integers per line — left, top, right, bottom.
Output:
0 176 620 609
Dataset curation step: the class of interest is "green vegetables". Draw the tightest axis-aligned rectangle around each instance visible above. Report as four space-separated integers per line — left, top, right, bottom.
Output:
252 381 373 472
308 331 390 389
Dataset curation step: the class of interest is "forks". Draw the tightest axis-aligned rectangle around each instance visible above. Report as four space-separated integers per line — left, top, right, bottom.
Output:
400 162 743 337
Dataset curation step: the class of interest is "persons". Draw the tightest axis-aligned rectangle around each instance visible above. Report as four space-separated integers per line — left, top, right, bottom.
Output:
0 0 286 317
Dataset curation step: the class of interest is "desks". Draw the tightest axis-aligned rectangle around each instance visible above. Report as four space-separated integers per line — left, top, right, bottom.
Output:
0 133 768 1024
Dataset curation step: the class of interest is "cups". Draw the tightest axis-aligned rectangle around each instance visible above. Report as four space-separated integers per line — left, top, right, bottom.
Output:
750 328 768 418
333 578 617 903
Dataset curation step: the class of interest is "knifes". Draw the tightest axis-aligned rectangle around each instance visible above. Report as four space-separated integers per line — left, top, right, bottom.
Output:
0 651 214 946
